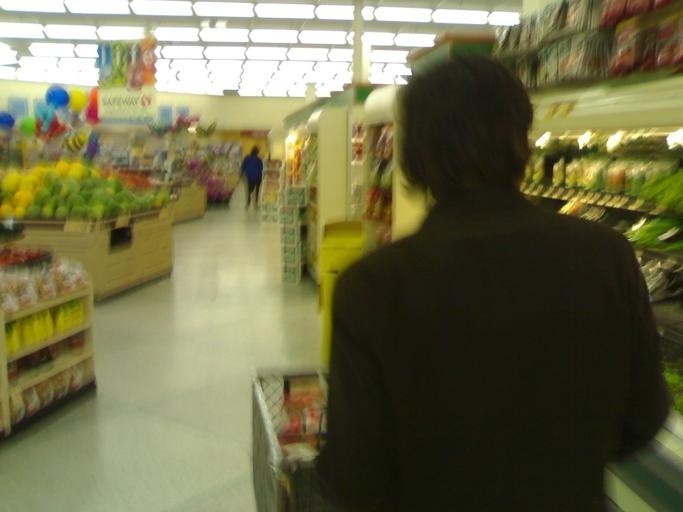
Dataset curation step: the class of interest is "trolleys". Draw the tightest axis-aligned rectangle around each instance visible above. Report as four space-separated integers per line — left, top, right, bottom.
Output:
253 373 319 512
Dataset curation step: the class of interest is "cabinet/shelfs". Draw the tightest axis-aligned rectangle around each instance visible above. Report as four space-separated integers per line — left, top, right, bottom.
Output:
0 274 97 438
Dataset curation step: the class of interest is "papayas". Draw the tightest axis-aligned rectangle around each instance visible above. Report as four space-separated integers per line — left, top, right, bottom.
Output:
0 160 162 221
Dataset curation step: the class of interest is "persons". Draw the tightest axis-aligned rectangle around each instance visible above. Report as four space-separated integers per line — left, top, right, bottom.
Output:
314 52 672 512
239 144 264 211
